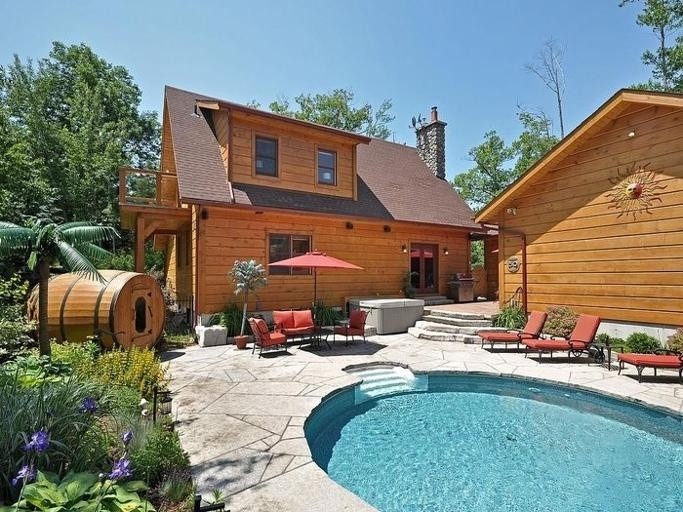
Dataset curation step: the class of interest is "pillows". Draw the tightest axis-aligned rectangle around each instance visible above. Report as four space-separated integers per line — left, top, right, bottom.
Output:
293 309 314 328
272 310 294 328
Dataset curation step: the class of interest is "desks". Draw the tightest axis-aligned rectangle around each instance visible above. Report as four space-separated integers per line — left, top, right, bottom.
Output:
587 343 625 371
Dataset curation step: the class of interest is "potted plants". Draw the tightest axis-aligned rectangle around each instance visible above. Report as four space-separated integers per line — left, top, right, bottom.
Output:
226 258 268 351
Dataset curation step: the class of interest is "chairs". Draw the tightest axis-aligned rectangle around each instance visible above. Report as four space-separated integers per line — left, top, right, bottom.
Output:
331 308 371 345
270 310 318 343
247 315 287 358
521 312 601 364
617 348 683 383
477 310 549 352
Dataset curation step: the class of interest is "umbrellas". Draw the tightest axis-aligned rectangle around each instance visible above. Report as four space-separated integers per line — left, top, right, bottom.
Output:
267 248 366 347
